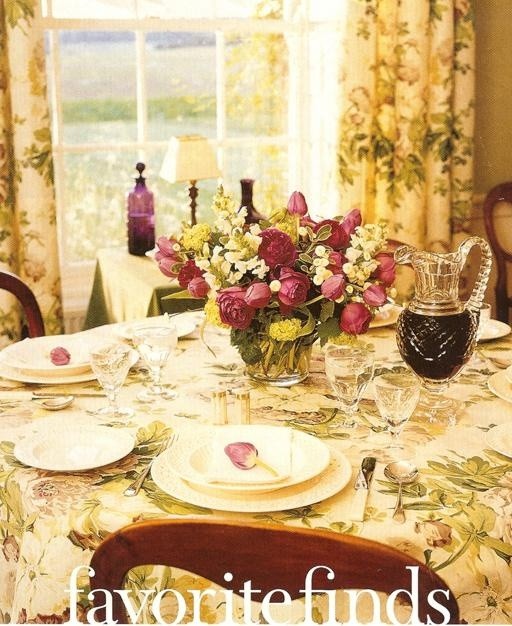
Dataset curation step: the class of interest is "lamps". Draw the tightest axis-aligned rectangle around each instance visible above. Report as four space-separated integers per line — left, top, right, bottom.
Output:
157 133 224 226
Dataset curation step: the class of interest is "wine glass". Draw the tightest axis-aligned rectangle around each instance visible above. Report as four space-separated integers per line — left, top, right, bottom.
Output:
372 372 423 455
128 321 179 403
84 339 128 415
323 346 376 425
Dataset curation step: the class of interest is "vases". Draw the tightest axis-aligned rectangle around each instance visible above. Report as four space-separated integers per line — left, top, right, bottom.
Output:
231 318 312 388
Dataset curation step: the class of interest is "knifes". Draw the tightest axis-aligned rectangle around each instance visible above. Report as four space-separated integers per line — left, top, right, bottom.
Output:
349 454 378 524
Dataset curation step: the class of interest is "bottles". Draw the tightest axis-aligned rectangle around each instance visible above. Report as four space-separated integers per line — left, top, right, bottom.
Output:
125 162 157 259
237 179 268 226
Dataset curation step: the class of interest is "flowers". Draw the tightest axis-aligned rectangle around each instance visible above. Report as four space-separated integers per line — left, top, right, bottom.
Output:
153 184 396 377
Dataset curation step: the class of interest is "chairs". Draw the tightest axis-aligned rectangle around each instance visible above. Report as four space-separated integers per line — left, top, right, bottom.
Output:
87 517 461 623
482 181 512 324
1 271 47 337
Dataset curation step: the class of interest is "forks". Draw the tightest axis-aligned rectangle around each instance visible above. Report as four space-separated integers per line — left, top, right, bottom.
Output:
123 433 179 497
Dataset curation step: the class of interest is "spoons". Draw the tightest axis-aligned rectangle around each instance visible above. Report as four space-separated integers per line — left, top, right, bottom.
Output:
0 393 75 419
493 356 509 366
382 459 421 525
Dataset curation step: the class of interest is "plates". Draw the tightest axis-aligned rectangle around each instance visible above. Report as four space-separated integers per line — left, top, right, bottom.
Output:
173 317 195 338
2 335 140 371
11 425 138 472
486 361 508 404
473 317 511 343
369 303 405 328
147 420 354 514
481 416 512 459
166 425 331 496
2 348 140 385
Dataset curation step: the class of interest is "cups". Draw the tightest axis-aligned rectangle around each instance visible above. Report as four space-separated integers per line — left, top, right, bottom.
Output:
466 301 491 330
